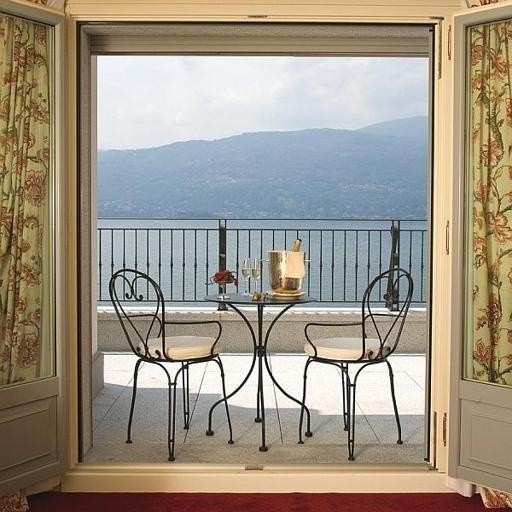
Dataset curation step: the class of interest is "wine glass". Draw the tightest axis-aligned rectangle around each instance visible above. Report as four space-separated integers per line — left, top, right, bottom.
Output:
241 258 262 296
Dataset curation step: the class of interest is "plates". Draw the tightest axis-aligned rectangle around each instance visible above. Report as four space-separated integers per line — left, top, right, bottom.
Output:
266 292 304 300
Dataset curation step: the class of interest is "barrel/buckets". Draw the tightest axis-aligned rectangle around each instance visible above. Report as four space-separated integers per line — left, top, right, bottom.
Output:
261 249 310 294
